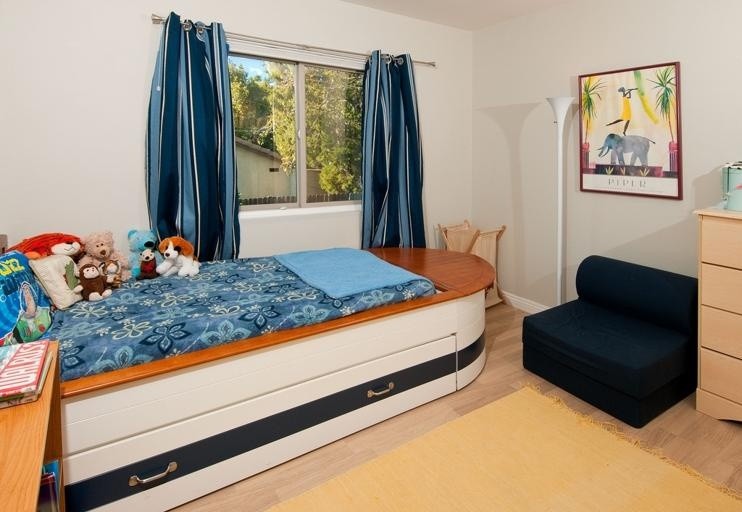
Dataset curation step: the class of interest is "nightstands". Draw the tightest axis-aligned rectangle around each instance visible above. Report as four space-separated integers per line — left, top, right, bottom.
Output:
0 343 63 512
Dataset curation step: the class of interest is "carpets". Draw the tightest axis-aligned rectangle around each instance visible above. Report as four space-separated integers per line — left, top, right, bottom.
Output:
262 386 742 512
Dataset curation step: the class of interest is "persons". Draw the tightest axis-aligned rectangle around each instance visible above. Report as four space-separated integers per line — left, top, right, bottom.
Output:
604 87 633 137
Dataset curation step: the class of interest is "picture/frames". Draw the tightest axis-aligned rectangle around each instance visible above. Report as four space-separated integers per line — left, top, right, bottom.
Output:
579 61 684 200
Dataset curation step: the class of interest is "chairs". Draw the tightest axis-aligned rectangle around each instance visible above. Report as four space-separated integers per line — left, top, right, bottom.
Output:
522 255 700 429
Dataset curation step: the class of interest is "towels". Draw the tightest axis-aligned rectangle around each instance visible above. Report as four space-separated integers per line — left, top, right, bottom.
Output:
272 247 436 300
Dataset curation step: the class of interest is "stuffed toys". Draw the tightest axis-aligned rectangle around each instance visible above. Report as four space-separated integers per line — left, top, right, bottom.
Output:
99 258 124 289
125 227 166 280
154 235 203 279
70 263 113 302
5 232 85 263
69 230 133 288
133 248 161 282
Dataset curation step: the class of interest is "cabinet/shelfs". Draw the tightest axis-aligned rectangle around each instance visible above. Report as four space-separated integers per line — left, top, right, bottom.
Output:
693 203 741 423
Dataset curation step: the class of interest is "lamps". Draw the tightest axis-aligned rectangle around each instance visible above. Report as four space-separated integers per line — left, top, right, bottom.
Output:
546 95 575 306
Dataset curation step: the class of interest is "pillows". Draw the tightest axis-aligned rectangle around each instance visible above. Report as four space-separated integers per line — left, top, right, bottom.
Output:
29 254 83 311
1 249 56 344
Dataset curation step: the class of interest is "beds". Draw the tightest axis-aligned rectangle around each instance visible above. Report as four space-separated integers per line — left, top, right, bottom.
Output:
0 234 496 512
